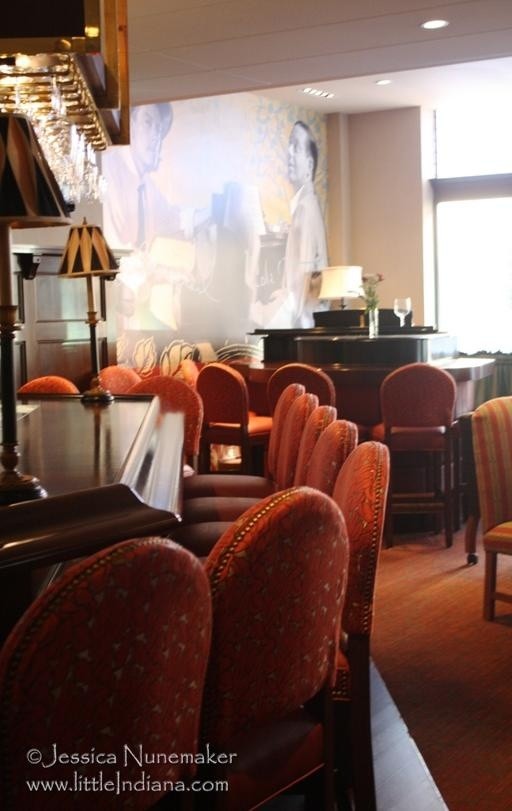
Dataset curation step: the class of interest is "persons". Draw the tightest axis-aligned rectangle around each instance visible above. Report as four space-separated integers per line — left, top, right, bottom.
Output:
103 102 189 331
250 121 330 330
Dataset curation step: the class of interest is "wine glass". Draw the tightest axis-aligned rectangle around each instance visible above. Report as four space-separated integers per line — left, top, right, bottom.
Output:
394 296 411 328
0 56 106 204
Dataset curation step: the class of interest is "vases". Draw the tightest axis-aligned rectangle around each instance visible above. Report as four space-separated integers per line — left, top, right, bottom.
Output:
368 309 379 339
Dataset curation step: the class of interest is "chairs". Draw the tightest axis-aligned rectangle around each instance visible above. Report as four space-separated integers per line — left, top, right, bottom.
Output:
179 358 495 548
0 377 390 811
471 396 512 621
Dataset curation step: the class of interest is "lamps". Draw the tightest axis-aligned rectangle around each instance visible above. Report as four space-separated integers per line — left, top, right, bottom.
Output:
0 113 73 505
318 265 365 310
57 217 121 404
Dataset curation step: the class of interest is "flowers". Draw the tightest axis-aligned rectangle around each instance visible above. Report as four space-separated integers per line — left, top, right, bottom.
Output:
358 273 386 335
